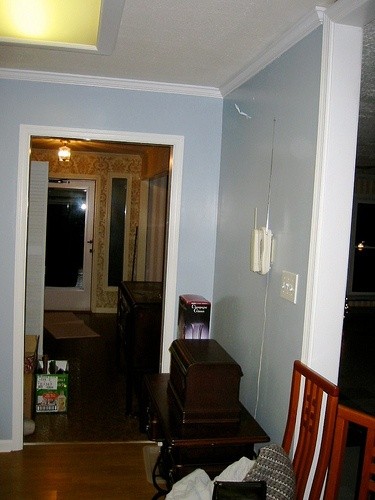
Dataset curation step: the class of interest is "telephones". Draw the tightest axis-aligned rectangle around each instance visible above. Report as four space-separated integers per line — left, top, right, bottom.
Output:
250 208 273 276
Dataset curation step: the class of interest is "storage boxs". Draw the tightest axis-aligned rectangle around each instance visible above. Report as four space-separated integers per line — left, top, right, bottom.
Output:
23 334 69 419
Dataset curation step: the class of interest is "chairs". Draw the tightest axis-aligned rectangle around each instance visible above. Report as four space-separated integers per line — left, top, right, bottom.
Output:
281 360 375 500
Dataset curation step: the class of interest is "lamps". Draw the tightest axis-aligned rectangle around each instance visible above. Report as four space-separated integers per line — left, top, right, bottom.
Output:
58 144 71 161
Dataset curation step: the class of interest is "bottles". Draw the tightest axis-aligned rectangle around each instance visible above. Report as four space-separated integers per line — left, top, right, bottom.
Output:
57 391 66 411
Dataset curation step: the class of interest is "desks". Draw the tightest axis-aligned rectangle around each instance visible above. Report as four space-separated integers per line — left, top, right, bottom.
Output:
44 312 100 398
117 279 162 421
151 373 271 488
